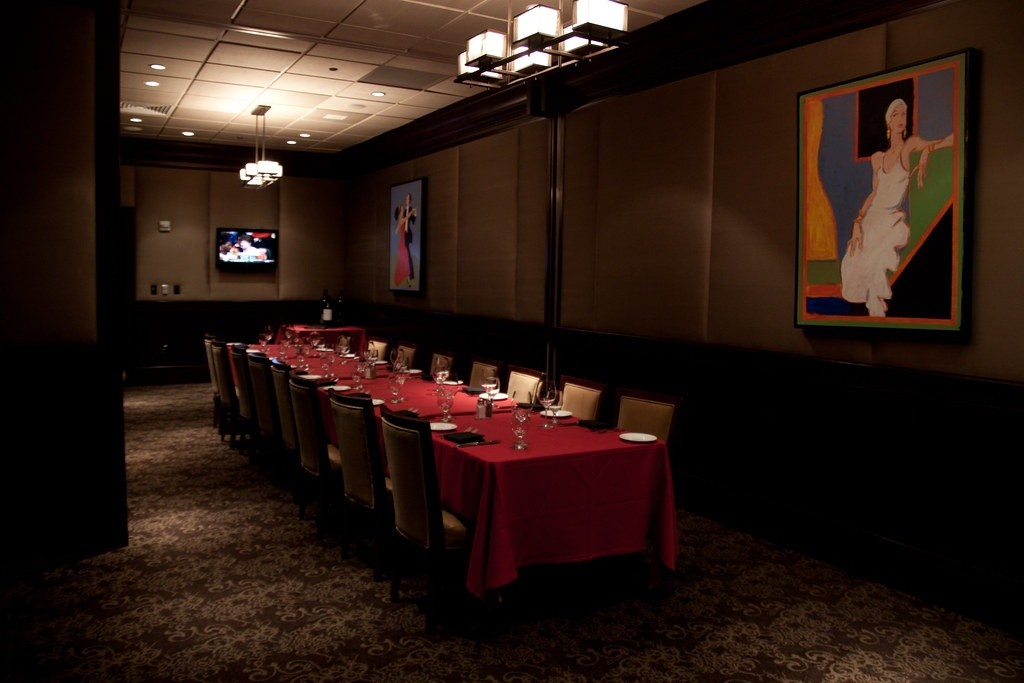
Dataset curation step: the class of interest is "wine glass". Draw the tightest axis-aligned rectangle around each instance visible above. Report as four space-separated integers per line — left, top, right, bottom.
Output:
537 380 554 429
550 390 564 429
511 390 531 433
258 321 499 421
511 413 528 451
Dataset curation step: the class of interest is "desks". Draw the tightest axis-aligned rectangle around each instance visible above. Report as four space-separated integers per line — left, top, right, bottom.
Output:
233 345 678 607
275 322 367 356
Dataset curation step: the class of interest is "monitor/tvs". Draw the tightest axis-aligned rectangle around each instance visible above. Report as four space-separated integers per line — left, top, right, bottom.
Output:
215 228 279 273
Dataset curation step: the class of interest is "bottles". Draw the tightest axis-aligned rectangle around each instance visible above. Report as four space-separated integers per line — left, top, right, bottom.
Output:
318 288 345 328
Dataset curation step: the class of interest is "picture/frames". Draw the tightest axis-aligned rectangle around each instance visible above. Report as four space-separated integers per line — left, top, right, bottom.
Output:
795 45 982 330
389 175 427 292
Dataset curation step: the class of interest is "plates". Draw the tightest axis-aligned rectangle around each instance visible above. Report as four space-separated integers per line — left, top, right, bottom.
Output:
540 409 574 417
479 393 509 400
619 432 658 443
227 341 462 405
430 423 457 431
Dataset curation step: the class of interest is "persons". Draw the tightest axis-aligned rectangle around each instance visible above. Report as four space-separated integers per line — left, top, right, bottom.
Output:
219 233 267 261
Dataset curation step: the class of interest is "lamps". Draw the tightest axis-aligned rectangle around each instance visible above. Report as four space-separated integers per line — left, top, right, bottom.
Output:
238 104 283 189
453 0 630 94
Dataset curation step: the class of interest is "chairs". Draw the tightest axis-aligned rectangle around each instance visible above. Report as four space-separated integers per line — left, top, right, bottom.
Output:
336 335 352 352
390 342 419 370
229 348 262 454
248 354 277 454
379 404 482 637
554 374 606 424
326 388 396 580
468 356 501 392
204 339 220 428
271 365 295 465
504 365 546 404
427 349 454 376
612 387 685 441
289 368 330 536
366 336 389 359
211 341 238 441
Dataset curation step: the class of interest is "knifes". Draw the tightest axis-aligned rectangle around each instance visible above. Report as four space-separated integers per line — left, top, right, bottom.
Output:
456 439 503 447
